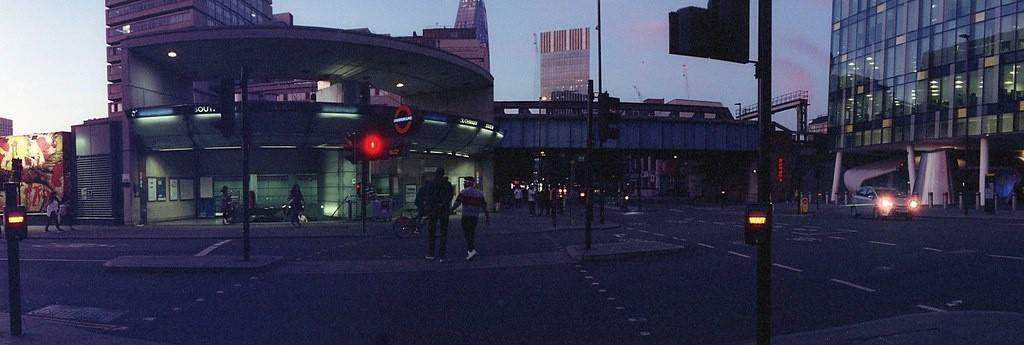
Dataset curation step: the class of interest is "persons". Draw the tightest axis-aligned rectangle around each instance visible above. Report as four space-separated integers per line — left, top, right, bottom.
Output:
220 186 234 225
414 166 490 263
43 191 77 232
288 181 306 227
514 184 551 217
494 185 501 213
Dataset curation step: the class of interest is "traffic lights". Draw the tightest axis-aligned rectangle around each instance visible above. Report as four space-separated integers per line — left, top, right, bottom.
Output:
598 90 621 143
364 187 376 201
5 207 24 229
356 183 361 197
362 125 379 161
207 77 237 139
343 130 360 164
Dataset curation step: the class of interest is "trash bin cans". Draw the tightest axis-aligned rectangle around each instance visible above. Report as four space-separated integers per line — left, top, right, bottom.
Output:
375 194 393 222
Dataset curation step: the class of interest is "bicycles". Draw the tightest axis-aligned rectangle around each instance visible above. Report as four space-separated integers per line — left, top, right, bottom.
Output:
392 208 442 239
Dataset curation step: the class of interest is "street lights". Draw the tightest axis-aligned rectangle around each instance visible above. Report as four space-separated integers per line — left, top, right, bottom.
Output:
537 96 548 216
959 33 972 216
734 103 741 207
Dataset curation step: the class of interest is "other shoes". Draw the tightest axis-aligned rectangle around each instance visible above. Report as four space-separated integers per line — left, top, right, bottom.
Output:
426 256 434 260
439 259 445 262
58 230 65 232
43 230 51 233
467 249 476 260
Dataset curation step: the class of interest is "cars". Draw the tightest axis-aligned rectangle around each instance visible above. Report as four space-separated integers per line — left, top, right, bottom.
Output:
850 185 920 222
